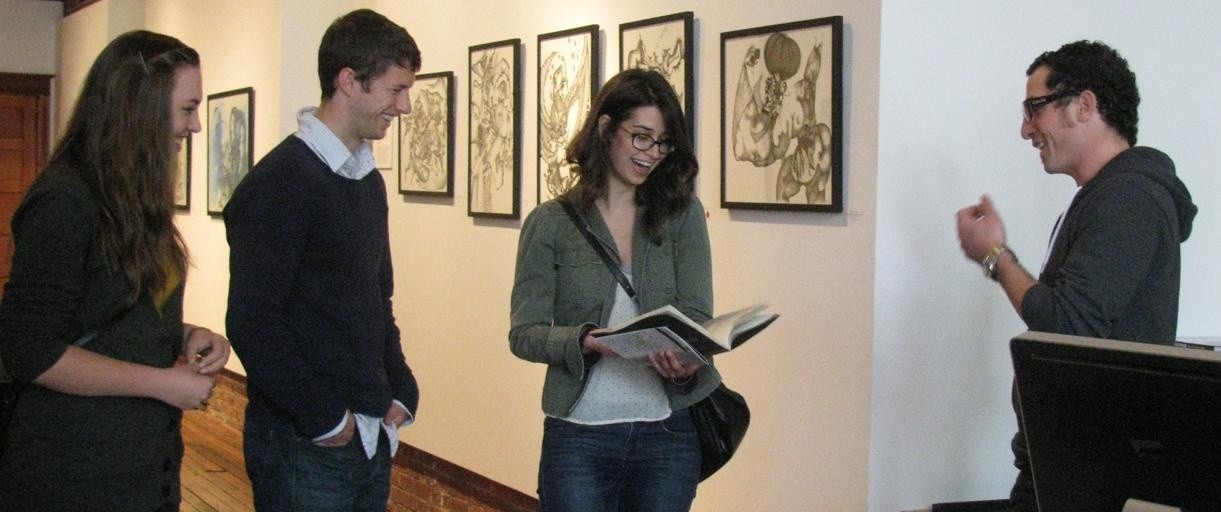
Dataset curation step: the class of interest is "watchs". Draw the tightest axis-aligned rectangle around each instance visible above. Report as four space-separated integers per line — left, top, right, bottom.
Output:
980 244 1018 280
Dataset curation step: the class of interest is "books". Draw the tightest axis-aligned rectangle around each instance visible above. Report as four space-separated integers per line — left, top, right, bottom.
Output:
587 302 781 357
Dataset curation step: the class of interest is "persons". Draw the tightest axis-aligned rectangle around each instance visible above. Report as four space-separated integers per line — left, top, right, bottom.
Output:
222 7 420 512
508 69 715 512
958 40 1199 512
2 30 233 511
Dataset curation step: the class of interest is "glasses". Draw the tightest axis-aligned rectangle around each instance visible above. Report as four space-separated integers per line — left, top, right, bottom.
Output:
619 126 677 155
1022 89 1081 124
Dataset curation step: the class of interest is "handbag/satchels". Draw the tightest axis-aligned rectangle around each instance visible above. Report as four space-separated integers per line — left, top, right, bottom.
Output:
688 382 750 483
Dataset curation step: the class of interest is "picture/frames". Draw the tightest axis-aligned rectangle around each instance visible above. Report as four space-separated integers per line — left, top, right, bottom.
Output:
173 131 192 210
620 11 693 154
399 70 454 196
537 24 600 205
207 87 254 216
721 14 843 213
468 39 520 219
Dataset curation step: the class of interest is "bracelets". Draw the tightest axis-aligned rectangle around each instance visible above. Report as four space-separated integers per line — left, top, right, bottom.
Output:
186 327 212 344
